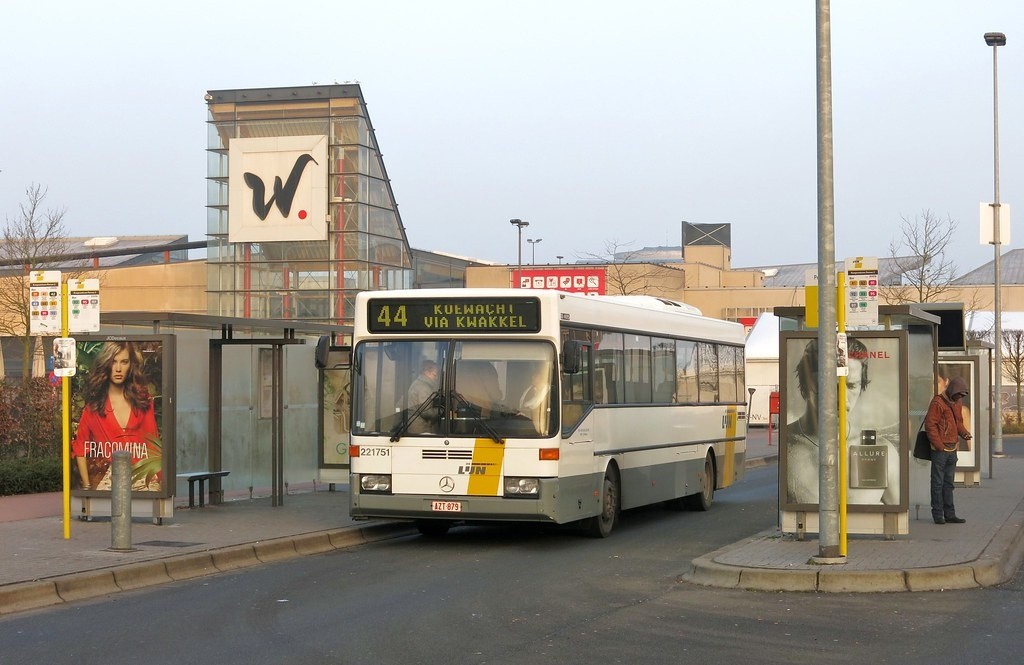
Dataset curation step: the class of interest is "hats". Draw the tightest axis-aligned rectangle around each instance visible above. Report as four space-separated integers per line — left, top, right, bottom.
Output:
959 392 967 395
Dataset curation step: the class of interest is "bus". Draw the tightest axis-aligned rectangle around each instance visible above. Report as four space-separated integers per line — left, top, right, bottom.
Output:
315 287 748 539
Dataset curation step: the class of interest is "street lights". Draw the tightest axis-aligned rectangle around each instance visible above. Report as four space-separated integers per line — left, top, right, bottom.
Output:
983 31 1007 458
510 219 530 288
526 239 543 265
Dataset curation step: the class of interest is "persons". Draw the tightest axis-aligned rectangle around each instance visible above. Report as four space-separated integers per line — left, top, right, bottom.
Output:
925 377 972 524
786 337 900 505
406 360 444 433
74 341 162 491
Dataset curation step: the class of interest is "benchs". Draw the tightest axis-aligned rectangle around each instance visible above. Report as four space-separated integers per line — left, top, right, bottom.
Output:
176 471 232 510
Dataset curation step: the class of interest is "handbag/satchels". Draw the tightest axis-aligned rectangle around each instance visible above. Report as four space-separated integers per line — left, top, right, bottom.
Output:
913 431 931 460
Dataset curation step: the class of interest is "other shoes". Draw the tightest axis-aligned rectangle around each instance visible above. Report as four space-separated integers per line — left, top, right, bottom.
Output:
945 516 965 523
934 517 945 523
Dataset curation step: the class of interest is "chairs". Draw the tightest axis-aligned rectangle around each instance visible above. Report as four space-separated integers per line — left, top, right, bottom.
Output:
612 379 738 404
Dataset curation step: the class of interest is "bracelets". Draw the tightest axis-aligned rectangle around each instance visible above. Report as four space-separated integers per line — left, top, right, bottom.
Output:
82 484 91 488
